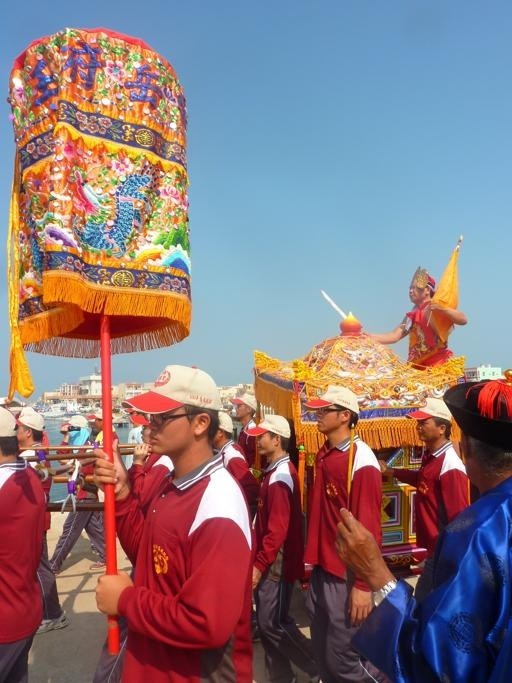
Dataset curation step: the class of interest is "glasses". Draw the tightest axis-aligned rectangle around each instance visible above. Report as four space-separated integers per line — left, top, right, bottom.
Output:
319 407 342 415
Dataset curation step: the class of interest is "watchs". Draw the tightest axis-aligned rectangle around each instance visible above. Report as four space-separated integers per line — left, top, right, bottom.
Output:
371 579 397 608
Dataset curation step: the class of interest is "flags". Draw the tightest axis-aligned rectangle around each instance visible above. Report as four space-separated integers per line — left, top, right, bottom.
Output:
422 244 462 348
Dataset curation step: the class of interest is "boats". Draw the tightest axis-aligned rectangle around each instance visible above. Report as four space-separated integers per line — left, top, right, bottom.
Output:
39 411 65 419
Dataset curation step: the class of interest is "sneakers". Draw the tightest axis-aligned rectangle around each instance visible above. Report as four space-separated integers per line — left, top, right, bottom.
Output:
36 611 71 634
89 562 106 570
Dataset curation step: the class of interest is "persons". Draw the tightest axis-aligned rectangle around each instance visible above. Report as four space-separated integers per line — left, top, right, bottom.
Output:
48 412 119 575
95 365 254 683
213 394 260 501
358 263 470 369
125 407 173 518
299 381 384 683
328 366 512 682
243 410 319 683
370 395 472 602
1 406 70 682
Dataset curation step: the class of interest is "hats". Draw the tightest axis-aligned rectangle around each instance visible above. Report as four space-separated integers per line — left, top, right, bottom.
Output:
120 364 291 439
0 406 46 438
443 370 512 451
404 397 451 421
87 409 102 420
303 386 359 415
